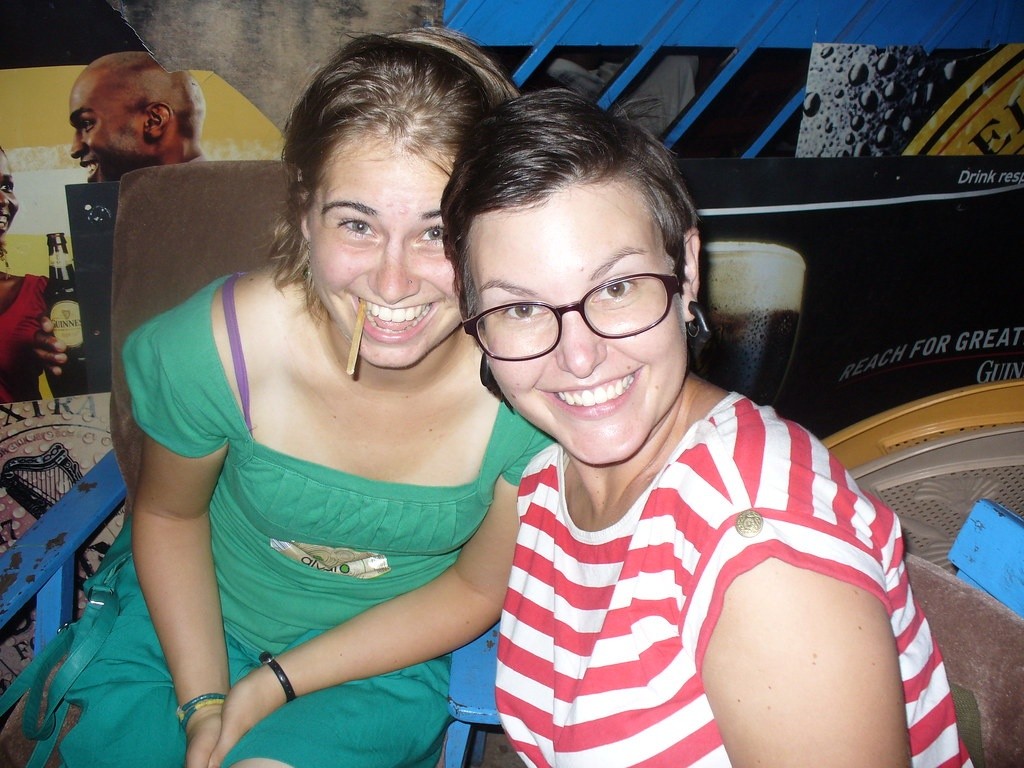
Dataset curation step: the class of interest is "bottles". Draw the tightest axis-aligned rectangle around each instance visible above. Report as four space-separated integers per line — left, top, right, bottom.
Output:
44 233 83 378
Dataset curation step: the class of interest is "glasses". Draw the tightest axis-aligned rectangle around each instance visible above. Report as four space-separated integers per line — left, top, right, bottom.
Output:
459 245 691 361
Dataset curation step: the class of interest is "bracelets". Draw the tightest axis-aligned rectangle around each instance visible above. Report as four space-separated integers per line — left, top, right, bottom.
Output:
258 652 296 703
177 693 225 731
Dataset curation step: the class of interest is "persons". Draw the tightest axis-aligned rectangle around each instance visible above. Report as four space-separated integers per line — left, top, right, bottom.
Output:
14 26 558 766
0 144 50 406
435 87 974 768
32 50 206 400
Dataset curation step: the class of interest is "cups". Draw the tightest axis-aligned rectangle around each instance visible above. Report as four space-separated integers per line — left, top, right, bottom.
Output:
685 249 805 407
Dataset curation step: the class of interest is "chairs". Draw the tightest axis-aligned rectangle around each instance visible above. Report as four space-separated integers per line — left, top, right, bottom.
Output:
903 552 1024 768
1 161 498 768
